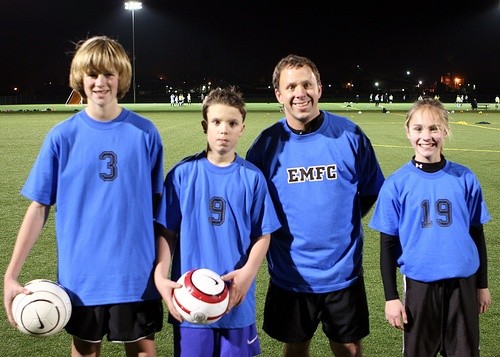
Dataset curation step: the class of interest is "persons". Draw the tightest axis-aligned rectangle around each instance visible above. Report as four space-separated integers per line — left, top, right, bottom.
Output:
434 95 440 101
154 85 282 357
169 92 191 107
245 54 385 357
471 99 477 111
418 96 423 102
369 93 393 108
4 37 164 357
368 99 492 357
455 94 468 109
495 97 499 107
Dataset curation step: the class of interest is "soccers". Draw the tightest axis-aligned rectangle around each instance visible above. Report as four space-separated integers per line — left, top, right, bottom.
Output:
173 268 230 326
10 278 73 338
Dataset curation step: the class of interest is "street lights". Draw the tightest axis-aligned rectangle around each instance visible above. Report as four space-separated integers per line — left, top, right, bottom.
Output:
124 1 142 103
